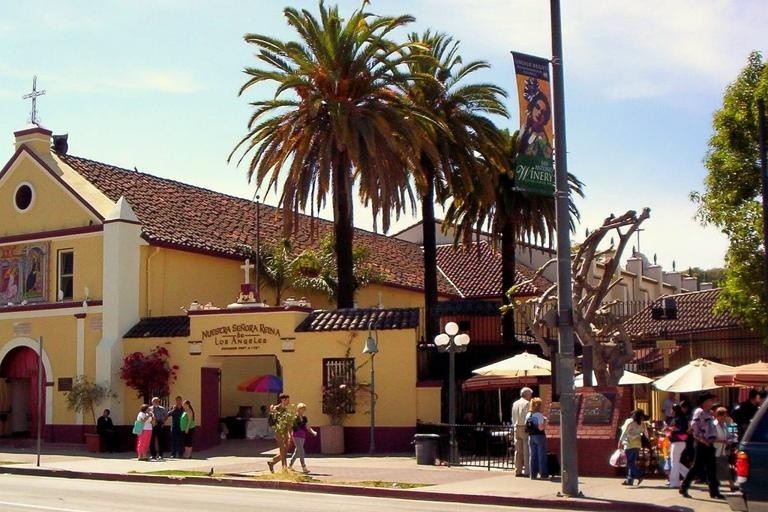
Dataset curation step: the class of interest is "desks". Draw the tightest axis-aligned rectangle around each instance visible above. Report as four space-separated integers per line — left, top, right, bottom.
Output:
234 416 269 441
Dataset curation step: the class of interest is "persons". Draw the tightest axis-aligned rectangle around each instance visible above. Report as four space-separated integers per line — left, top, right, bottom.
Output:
618 389 768 500
95 409 113 453
516 92 551 158
511 386 533 478
288 402 317 473
267 393 293 474
525 397 549 480
136 396 195 461
259 405 268 418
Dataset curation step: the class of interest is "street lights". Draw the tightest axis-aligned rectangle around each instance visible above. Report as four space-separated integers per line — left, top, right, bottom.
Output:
362 319 380 455
433 322 471 461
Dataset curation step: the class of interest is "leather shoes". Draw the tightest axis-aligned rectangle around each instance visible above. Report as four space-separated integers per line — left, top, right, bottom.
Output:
679 488 692 498
710 492 725 499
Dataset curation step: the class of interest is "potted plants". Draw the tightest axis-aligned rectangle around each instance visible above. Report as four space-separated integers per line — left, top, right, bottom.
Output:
312 328 382 458
61 374 123 454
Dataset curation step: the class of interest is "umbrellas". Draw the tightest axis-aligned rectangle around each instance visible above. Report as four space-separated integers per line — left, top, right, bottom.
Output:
572 364 654 390
651 358 736 393
236 374 283 400
735 360 768 371
472 351 552 387
461 374 538 422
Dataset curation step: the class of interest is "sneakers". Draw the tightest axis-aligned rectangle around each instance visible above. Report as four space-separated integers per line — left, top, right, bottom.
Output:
637 474 644 485
515 474 529 478
137 453 193 461
267 461 311 474
731 486 739 492
530 473 553 480
621 479 633 486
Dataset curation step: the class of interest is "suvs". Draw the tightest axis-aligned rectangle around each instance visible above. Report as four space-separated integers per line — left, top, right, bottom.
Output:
717 396 768 512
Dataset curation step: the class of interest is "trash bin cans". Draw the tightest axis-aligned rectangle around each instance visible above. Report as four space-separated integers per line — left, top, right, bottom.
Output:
411 434 440 465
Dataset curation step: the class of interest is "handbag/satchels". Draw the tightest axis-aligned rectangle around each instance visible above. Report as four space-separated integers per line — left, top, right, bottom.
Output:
715 456 732 481
524 412 546 436
132 420 143 435
640 425 653 448
268 414 279 427
676 433 688 440
679 446 695 468
